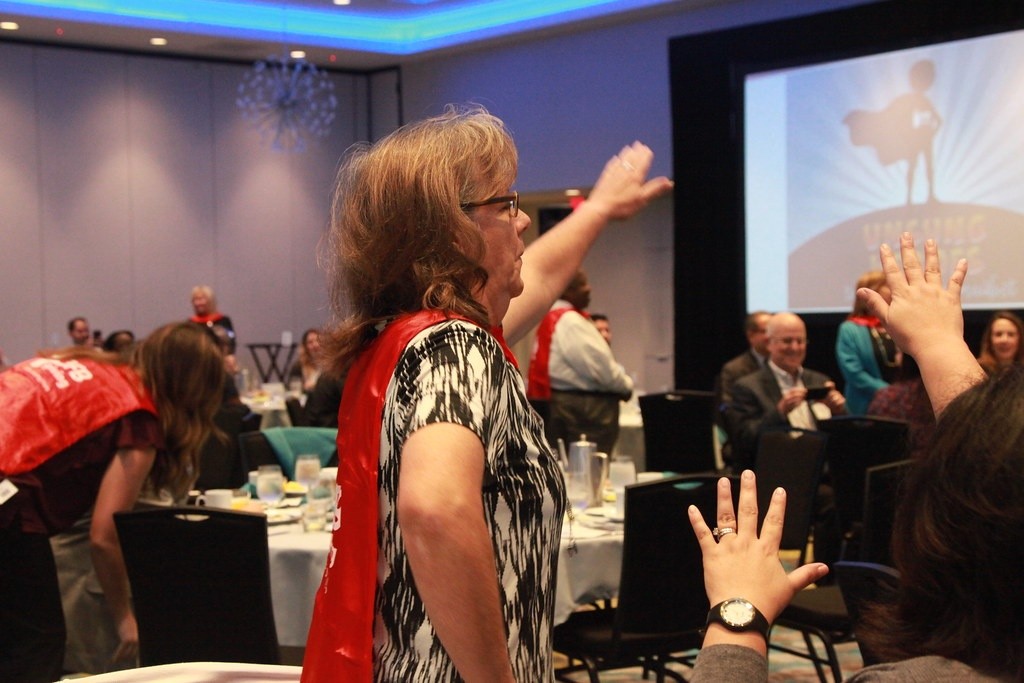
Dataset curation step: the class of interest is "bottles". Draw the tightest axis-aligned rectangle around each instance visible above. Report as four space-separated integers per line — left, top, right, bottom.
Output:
241 369 249 392
610 455 636 487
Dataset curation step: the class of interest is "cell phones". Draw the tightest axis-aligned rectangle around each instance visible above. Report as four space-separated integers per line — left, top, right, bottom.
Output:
802 387 831 401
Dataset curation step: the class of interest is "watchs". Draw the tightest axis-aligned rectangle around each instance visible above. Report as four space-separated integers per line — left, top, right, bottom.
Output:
706 598 770 648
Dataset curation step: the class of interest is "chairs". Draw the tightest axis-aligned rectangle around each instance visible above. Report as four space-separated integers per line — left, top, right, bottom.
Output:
23 338 1024 683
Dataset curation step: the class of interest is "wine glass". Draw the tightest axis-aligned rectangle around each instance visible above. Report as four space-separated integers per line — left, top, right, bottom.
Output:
295 454 321 502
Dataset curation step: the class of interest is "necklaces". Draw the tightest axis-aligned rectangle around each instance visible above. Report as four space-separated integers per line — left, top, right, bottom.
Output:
870 327 903 366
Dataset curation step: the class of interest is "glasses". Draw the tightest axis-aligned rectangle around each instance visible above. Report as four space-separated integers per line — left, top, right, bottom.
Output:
460 190 519 217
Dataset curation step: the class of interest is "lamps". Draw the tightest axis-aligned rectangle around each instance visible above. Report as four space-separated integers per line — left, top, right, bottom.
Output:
234 0 337 149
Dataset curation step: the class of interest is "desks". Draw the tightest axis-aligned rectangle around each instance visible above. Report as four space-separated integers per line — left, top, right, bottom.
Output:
237 382 305 413
140 467 675 643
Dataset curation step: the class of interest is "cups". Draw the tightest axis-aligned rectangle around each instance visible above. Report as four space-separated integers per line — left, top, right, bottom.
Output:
248 472 259 499
301 504 326 532
195 489 232 510
256 465 283 501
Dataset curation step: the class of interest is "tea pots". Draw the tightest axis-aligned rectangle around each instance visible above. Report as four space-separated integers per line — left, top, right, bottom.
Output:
557 434 607 509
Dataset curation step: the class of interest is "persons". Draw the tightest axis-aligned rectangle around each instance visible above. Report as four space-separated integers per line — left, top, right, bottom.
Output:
718 310 852 476
188 284 236 368
975 310 1024 377
69 317 133 354
285 327 350 429
0 321 227 683
526 268 634 460
687 231 1024 683
836 270 920 418
318 102 672 683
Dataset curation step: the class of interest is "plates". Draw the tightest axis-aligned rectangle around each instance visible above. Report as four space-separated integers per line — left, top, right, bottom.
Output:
285 492 306 497
606 512 624 522
264 509 302 523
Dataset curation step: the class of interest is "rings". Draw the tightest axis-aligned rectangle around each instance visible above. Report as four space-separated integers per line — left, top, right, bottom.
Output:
623 161 632 170
712 527 736 541
